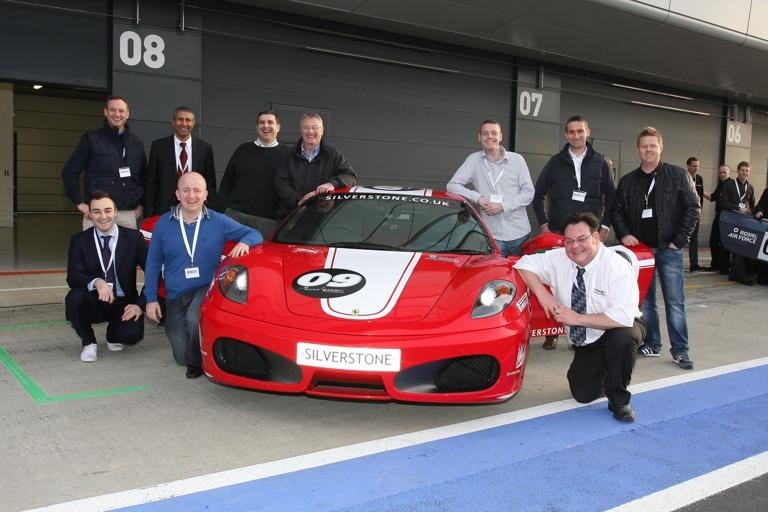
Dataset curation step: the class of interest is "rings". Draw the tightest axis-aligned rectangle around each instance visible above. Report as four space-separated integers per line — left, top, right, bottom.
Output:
555 307 560 314
105 295 109 298
128 312 132 316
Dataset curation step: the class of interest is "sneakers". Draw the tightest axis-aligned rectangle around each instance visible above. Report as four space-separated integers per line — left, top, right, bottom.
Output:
638 345 662 357
672 353 693 367
107 342 123 352
81 344 97 362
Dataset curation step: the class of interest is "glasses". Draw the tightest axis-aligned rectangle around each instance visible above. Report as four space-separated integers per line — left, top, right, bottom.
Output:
564 232 593 248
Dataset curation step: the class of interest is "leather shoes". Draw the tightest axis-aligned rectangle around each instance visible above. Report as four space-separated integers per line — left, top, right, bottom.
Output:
608 401 635 422
542 336 558 350
692 262 728 274
186 365 202 378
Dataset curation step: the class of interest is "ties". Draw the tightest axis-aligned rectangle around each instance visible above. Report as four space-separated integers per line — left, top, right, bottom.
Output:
101 239 111 272
570 270 587 345
177 143 189 176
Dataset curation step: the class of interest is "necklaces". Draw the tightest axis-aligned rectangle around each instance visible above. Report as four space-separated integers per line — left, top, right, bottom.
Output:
175 204 205 223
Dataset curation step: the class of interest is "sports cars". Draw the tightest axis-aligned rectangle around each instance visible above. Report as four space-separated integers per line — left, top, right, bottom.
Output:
136 184 660 406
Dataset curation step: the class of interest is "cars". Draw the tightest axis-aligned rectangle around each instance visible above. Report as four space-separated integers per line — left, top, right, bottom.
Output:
717 207 768 267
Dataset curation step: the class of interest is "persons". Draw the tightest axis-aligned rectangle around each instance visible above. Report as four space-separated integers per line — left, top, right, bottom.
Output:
717 161 757 287
699 164 732 276
58 189 150 365
509 210 649 421
58 96 146 233
445 119 535 255
753 186 768 220
216 110 290 237
142 170 264 380
684 156 705 273
588 142 613 172
530 115 618 349
271 111 358 218
611 126 702 373
146 105 217 214
